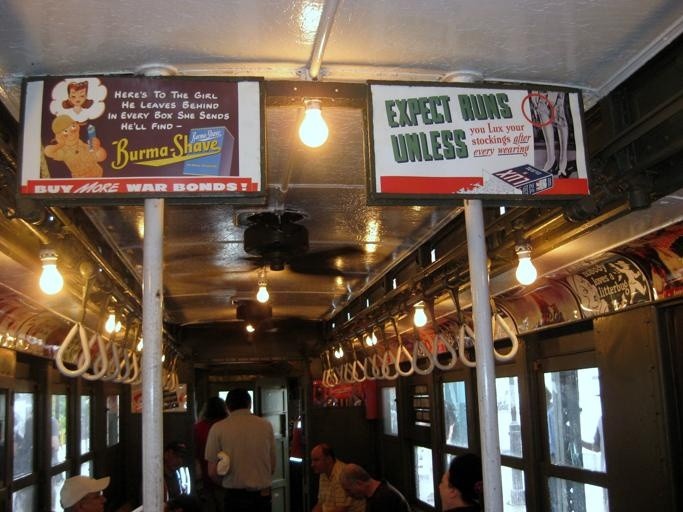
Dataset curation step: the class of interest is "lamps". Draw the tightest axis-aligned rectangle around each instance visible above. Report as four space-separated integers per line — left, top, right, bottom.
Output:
37 232 64 295
254 267 269 303
408 299 429 329
511 240 537 288
297 93 330 148
102 301 121 334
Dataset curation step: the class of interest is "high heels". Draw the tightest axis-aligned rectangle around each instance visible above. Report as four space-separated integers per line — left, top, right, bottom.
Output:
542 160 572 179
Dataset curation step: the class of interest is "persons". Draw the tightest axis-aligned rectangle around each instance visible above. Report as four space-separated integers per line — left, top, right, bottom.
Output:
530 90 569 179
162 441 194 511
544 386 563 512
437 451 486 511
194 396 230 511
203 387 277 510
340 463 412 511
1 415 64 512
581 393 609 512
310 443 366 512
